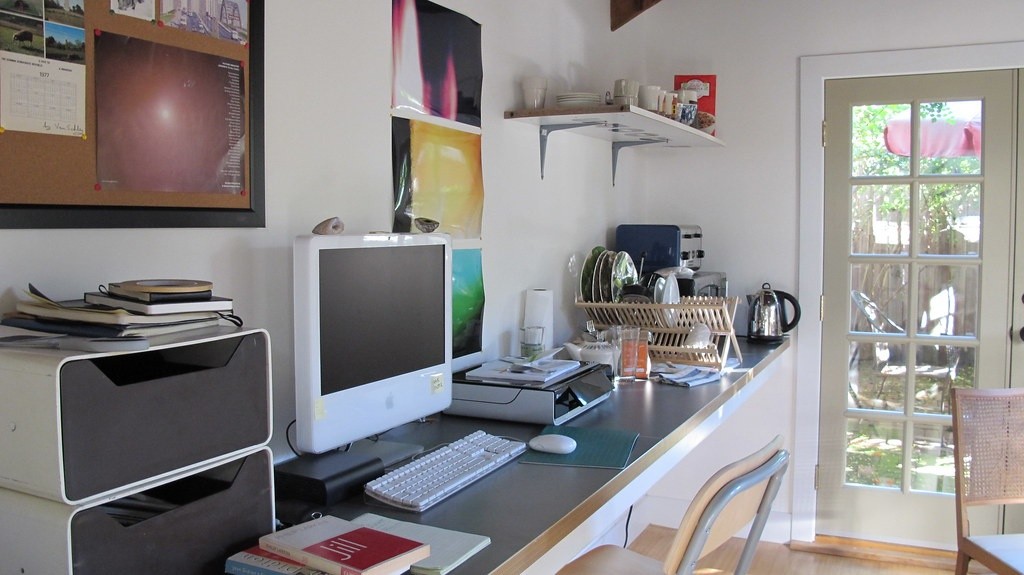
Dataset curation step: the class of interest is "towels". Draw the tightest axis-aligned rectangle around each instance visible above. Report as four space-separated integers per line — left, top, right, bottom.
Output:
653 366 723 388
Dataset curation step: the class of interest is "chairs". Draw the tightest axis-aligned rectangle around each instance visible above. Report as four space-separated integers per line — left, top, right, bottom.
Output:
852 290 964 412
951 386 1024 574
555 431 793 575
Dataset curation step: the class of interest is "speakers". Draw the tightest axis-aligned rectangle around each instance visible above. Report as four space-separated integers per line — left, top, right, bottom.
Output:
0 446 276 575
0 325 273 506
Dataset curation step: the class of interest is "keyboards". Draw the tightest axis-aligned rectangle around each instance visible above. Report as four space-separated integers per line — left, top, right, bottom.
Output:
364 430 527 513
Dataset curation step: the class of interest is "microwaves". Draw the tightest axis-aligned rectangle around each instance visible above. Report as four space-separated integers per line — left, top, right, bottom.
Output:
655 273 726 297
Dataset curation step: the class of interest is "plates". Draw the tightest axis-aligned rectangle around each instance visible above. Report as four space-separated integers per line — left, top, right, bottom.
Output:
555 93 601 105
580 245 679 327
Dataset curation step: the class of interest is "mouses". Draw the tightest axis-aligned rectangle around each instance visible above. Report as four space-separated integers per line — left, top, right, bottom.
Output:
529 435 576 454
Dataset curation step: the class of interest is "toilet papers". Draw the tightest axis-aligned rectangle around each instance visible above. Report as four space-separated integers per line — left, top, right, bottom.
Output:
523 288 553 361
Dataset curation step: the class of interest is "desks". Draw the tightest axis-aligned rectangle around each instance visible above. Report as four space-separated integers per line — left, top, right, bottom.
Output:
270 336 791 575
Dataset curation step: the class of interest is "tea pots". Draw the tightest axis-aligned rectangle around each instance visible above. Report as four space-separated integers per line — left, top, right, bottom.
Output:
563 340 620 368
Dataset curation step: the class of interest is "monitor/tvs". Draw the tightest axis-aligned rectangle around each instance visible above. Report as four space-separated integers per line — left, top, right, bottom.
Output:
289 233 452 469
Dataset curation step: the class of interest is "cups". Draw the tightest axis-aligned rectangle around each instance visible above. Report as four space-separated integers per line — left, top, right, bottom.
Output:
613 79 697 127
613 325 640 386
523 77 547 109
519 327 545 355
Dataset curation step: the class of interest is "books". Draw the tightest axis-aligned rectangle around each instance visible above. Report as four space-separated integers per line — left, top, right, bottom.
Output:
0 278 234 339
225 513 492 575
465 355 581 382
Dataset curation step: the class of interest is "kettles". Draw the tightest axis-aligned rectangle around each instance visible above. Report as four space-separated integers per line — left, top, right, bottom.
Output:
745 283 801 341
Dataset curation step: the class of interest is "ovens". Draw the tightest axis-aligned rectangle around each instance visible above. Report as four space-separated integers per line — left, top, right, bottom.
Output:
616 223 704 271
